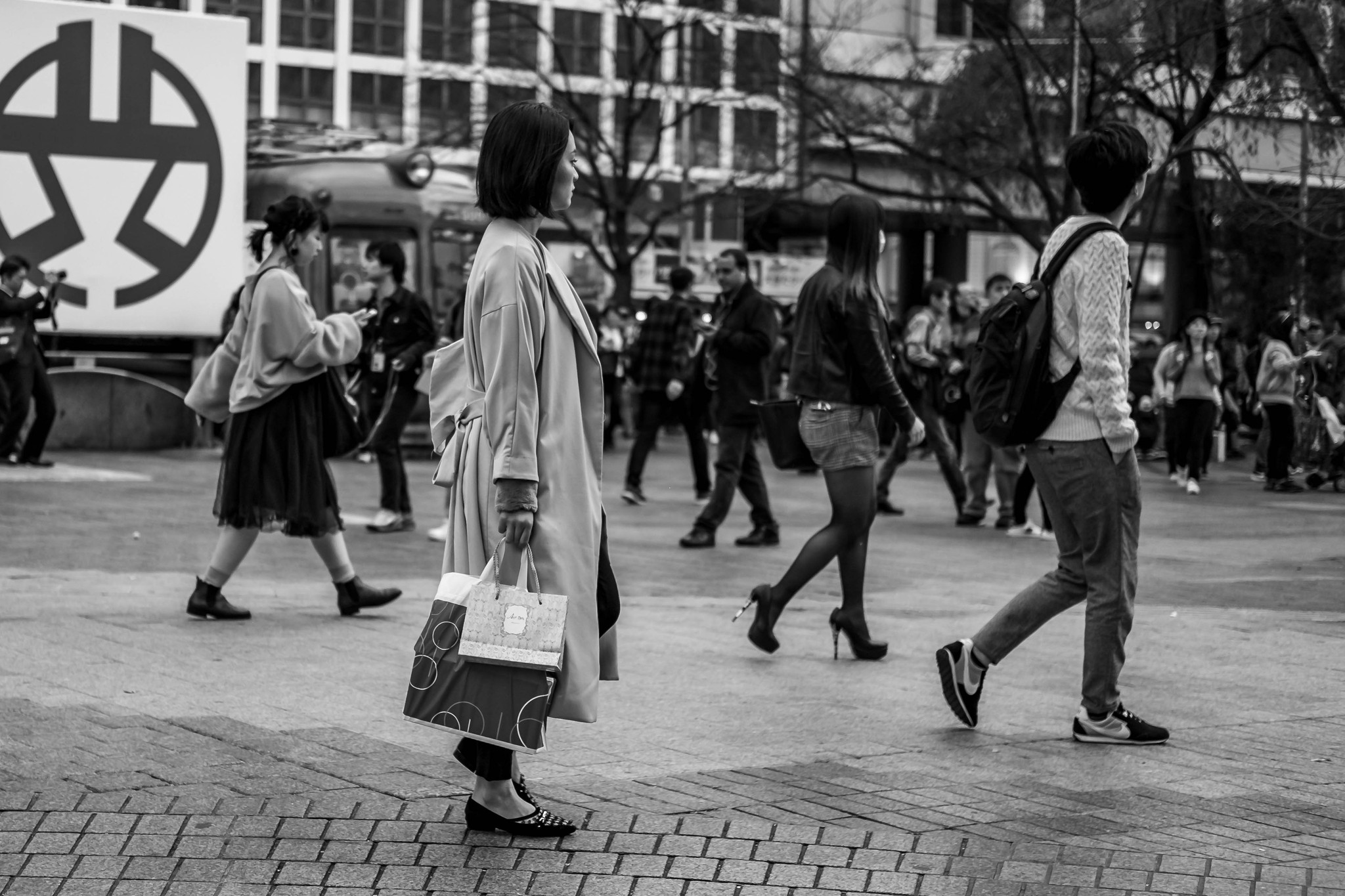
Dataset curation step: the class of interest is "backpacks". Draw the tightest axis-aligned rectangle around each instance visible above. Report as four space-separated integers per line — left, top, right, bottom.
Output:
970 223 1132 446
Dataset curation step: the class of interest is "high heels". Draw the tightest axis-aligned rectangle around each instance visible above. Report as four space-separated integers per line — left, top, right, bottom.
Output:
830 607 887 659
732 584 779 654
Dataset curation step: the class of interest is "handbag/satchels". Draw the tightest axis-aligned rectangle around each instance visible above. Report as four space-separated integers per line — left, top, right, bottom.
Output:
326 368 370 458
458 536 567 672
404 536 558 754
752 396 815 468
1317 396 1345 449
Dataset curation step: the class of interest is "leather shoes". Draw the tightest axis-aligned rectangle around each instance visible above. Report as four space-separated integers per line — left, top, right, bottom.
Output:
187 578 251 620
334 575 401 615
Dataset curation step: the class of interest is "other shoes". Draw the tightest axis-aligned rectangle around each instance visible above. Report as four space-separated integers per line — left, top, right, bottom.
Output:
514 773 543 808
879 499 1056 542
680 528 715 547
1136 447 1314 496
736 523 779 545
694 489 714 505
0 448 53 469
465 797 576 836
623 483 646 505
428 518 447 542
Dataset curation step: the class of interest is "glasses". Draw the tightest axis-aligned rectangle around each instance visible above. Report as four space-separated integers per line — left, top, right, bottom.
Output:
715 264 744 275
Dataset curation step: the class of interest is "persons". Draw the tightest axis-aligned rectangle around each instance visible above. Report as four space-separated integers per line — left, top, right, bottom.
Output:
920 123 1172 745
0 190 1344 662
407 104 622 843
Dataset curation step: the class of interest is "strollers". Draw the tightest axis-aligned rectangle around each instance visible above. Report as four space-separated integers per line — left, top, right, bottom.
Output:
1298 360 1345 493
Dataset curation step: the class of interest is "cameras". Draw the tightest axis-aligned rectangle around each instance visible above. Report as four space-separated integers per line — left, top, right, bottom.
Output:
56 271 67 278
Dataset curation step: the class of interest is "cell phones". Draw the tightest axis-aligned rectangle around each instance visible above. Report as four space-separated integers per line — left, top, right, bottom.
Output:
356 310 377 323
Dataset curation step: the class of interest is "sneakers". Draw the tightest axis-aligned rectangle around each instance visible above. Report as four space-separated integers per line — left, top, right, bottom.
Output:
936 638 989 726
366 506 414 531
1072 706 1169 744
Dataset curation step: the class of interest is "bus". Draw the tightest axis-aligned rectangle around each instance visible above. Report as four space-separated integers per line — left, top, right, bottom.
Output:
248 149 490 452
